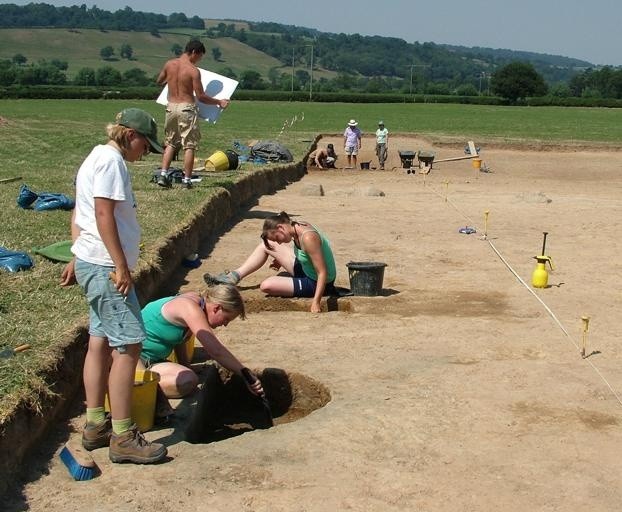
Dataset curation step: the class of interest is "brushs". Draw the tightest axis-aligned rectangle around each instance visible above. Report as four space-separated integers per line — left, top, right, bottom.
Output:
56 426 96 482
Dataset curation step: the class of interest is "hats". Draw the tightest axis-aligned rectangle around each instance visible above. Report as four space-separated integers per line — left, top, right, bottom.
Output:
378 121 384 125
348 119 359 126
117 107 166 155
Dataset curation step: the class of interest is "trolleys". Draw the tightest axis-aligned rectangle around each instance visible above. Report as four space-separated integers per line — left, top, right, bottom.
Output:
399 150 416 166
418 149 435 169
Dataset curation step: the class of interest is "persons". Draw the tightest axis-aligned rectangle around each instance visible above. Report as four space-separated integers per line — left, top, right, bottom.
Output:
59 108 166 464
107 283 266 400
204 212 336 313
156 39 231 189
308 120 388 171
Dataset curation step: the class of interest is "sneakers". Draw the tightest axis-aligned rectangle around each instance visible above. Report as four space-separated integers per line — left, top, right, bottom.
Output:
82 412 114 451
109 425 168 464
158 174 172 188
203 270 241 286
182 178 192 185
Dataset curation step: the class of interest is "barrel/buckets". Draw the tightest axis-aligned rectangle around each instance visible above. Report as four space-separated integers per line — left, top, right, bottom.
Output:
205 151 229 172
165 333 196 366
473 159 482 168
105 370 161 434
360 162 369 169
346 261 387 296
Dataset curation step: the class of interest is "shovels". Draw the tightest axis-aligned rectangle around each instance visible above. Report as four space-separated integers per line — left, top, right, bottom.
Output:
240 367 274 427
0 344 30 359
